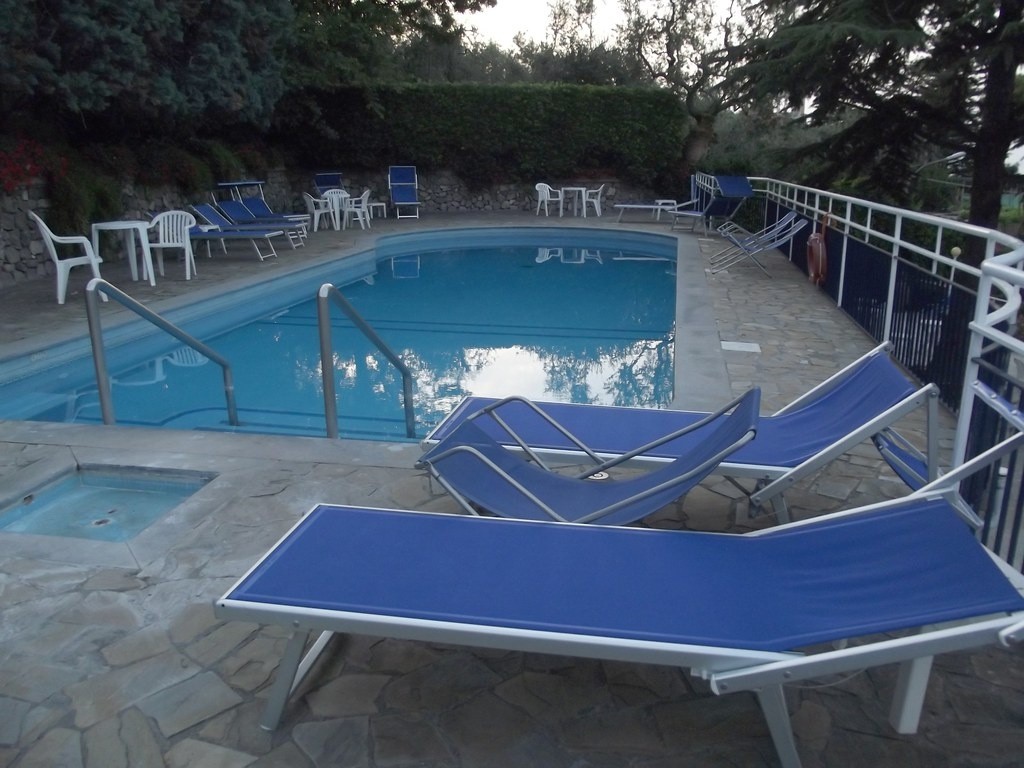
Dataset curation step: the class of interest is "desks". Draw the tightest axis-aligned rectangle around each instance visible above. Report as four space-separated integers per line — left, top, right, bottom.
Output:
561 187 586 218
198 224 227 257
560 247 585 263
354 203 388 219
92 218 158 289
650 200 680 222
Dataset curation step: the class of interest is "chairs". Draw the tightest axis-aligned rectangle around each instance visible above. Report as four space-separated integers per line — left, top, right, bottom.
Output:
142 209 197 281
613 251 670 261
364 275 376 284
392 255 421 278
27 210 109 304
218 343 1024 768
387 164 420 218
536 247 561 262
583 249 603 265
613 173 807 277
147 172 371 260
535 182 564 217
581 183 605 217
63 341 213 423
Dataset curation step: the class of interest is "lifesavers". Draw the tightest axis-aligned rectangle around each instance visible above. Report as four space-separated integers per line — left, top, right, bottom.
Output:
806 232 828 284
